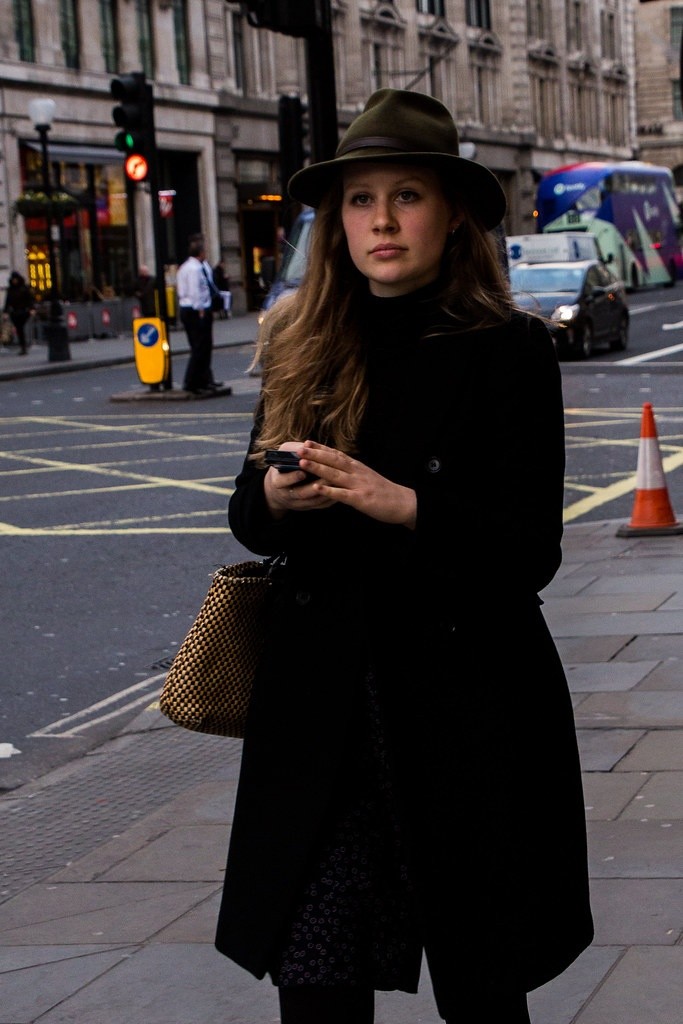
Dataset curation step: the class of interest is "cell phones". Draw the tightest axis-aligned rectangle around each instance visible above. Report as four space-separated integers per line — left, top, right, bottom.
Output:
262 449 320 487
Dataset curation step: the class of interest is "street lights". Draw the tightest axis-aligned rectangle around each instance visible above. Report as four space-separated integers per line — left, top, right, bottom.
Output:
27 91 72 361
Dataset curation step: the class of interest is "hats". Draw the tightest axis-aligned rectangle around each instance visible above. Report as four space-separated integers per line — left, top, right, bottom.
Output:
288 89 507 234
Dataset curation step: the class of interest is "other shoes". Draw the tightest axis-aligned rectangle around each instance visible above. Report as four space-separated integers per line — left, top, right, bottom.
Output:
184 377 224 390
18 349 27 356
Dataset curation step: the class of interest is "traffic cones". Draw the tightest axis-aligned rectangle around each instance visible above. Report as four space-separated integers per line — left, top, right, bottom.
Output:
616 401 683 539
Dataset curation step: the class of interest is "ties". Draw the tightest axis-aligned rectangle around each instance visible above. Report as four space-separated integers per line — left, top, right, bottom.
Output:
202 266 212 294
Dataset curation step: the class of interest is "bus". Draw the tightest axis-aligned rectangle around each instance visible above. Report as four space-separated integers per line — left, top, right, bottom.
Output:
532 158 683 293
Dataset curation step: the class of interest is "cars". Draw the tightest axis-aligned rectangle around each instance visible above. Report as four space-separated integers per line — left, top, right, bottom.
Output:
502 226 631 357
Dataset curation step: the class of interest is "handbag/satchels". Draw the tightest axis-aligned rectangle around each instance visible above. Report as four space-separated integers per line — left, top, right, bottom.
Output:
159 559 289 739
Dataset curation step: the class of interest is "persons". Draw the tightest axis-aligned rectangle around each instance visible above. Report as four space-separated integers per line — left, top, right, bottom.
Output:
3 271 36 356
174 234 227 396
214 88 595 1024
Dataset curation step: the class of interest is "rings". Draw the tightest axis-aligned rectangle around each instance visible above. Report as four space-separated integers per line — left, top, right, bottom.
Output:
289 487 295 501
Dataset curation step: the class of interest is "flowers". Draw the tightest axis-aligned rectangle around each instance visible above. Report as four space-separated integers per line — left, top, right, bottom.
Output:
6 187 84 233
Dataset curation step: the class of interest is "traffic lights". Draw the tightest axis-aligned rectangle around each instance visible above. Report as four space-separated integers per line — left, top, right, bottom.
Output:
108 70 157 155
277 95 309 179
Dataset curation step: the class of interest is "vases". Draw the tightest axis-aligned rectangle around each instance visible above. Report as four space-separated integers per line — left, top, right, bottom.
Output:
16 200 77 221
